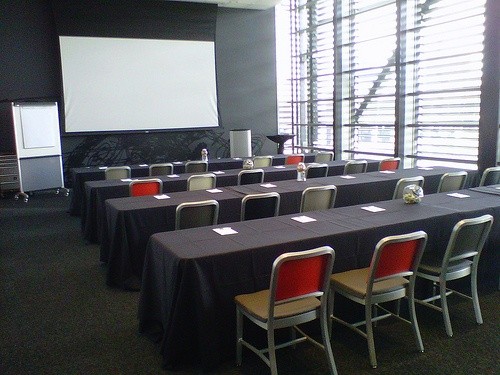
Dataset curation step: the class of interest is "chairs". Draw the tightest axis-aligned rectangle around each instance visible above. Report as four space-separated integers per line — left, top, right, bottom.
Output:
105 153 500 375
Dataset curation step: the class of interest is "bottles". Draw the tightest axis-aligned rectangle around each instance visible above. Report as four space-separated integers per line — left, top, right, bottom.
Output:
297 162 307 181
201 148 209 162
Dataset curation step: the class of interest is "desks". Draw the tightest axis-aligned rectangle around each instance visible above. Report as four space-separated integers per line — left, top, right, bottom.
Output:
100 165 479 293
67 154 316 216
80 159 380 240
139 183 500 375
